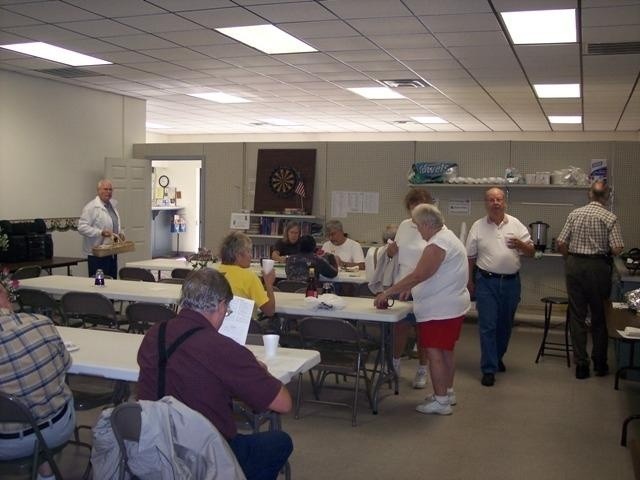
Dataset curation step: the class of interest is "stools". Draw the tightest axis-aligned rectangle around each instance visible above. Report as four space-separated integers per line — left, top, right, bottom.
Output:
535 296 575 367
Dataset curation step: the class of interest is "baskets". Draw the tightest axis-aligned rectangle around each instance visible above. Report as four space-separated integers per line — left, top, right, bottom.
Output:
93 232 135 258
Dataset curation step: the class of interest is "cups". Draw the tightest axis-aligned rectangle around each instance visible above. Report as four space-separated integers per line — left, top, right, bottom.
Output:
505 234 514 246
262 258 275 274
262 335 281 360
375 292 394 309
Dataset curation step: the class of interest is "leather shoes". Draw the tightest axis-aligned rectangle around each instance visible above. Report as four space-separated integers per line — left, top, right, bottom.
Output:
482 373 495 386
594 360 609 372
576 367 590 379
497 359 506 373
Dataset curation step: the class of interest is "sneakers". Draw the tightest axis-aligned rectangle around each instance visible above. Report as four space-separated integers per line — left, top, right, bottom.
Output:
426 390 456 406
416 400 453 415
412 370 429 389
381 365 400 381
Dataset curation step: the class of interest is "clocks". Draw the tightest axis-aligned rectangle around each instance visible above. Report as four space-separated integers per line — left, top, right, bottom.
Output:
159 175 168 188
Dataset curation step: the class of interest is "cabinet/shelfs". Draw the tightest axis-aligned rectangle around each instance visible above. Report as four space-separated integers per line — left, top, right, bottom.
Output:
231 212 322 261
406 184 613 326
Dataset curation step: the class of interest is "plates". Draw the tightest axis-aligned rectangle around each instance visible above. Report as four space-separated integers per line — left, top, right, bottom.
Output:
615 328 640 340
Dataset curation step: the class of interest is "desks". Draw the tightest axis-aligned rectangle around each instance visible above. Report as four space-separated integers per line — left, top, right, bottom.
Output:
607 254 640 447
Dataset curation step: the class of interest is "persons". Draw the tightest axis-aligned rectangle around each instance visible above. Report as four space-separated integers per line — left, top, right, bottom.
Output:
137 267 294 480
382 190 447 389
556 181 625 380
374 203 471 417
464 188 535 388
76 178 125 315
269 220 416 359
213 231 282 348
0 281 76 480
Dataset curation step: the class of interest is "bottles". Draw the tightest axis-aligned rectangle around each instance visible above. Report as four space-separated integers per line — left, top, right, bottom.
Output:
322 283 331 294
306 268 318 299
95 268 104 285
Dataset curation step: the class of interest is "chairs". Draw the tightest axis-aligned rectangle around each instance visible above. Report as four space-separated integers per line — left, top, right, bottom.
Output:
1 252 413 480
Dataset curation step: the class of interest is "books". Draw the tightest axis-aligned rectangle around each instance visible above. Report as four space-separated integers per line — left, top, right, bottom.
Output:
241 217 311 262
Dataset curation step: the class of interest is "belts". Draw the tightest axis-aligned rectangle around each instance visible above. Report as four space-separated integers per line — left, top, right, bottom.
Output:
0 403 68 440
475 265 520 278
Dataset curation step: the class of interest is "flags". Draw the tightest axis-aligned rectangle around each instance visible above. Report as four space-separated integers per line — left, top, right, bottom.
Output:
295 178 306 197
